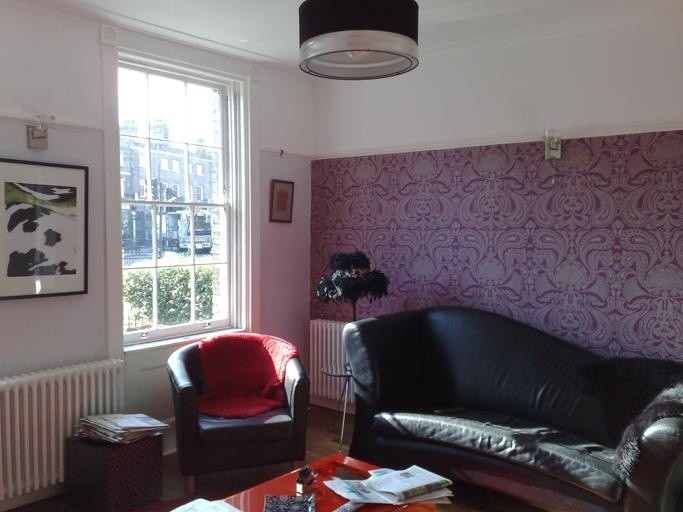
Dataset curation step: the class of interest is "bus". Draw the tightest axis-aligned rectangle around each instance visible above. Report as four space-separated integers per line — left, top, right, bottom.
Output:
165 210 212 252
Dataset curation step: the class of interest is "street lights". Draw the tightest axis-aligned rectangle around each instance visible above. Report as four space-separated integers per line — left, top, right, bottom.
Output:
128 201 137 242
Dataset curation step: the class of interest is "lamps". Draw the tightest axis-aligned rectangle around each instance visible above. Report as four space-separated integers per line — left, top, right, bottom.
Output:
297 1 421 80
544 128 563 184
25 113 55 151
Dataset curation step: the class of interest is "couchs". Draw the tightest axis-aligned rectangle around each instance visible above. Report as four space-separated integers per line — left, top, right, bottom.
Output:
165 333 311 493
340 306 683 511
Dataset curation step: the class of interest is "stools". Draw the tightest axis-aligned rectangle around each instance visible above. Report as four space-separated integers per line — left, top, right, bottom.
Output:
67 432 165 510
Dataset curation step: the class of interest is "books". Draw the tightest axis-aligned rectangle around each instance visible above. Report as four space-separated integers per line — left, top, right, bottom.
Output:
79 408 168 447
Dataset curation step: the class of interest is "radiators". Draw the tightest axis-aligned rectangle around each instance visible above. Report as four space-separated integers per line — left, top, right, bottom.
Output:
308 321 360 409
0 357 126 505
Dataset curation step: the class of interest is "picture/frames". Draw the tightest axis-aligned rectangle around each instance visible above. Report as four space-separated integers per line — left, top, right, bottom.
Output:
268 177 295 225
0 156 90 301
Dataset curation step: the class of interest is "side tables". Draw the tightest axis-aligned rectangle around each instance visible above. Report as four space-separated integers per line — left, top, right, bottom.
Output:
321 362 355 445
